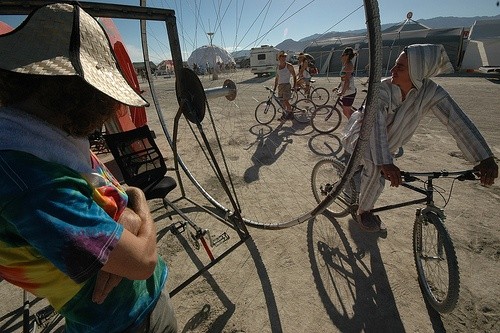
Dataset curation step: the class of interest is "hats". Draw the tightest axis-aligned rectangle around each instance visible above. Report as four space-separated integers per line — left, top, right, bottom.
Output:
277 52 288 58
0 0 150 108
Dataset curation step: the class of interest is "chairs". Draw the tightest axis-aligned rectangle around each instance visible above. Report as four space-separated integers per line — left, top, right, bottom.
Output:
103 124 179 197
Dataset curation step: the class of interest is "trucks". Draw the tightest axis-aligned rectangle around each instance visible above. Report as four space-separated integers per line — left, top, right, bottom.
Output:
250 45 279 76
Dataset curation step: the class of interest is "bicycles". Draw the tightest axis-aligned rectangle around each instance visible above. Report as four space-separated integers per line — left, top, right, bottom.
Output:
311 157 487 314
288 76 330 107
360 82 367 92
254 87 317 124
310 89 371 135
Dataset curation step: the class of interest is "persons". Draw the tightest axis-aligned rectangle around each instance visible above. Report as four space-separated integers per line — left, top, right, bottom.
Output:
0 3 176 333
273 50 296 121
339 44 498 233
296 54 311 98
332 47 357 119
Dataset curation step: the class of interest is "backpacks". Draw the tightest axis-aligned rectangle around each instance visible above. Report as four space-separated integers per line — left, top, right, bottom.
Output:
303 58 318 75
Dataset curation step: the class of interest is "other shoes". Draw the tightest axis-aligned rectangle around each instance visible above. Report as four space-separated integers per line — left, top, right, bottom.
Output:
277 112 293 120
355 211 381 233
376 215 387 233
